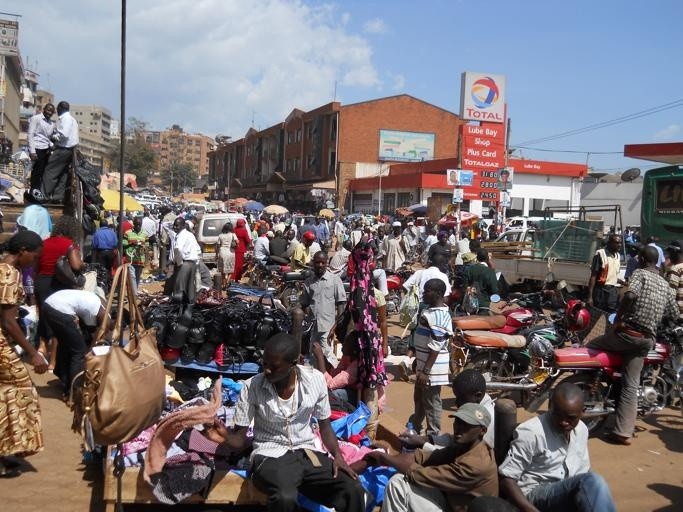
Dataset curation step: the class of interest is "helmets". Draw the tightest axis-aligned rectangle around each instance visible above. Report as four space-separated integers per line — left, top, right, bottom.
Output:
303 232 316 241
565 300 591 330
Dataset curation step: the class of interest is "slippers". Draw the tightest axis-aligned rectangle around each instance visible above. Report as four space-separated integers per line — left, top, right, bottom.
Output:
36 350 54 374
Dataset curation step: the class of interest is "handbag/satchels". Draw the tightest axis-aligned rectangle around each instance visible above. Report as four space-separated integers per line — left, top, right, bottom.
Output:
86 263 314 368
462 285 481 314
56 255 86 288
67 325 166 447
400 285 419 329
333 311 351 342
74 150 105 233
231 240 238 248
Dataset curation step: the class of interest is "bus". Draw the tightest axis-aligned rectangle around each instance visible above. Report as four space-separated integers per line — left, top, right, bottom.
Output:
639 166 683 249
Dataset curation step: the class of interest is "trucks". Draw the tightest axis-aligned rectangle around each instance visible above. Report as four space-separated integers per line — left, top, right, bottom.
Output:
488 216 627 295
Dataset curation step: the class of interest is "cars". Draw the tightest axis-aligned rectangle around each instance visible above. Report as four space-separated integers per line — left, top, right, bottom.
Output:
134 195 169 211
186 200 327 266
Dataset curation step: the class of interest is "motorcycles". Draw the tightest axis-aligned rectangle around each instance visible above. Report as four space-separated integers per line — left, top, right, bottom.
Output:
448 280 682 435
244 254 413 315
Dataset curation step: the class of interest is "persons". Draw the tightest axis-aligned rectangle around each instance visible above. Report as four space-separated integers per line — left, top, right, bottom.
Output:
212 333 365 512
497 383 618 512
362 402 499 512
40 101 79 205
584 246 680 446
12 199 683 416
401 279 453 439
0 230 48 477
398 371 497 452
447 171 459 185
26 103 55 190
497 170 512 188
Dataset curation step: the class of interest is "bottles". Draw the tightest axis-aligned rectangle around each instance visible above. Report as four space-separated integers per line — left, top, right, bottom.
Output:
401 422 417 456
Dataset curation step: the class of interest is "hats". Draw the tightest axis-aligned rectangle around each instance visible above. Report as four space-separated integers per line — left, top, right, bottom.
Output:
447 403 491 428
265 231 276 238
237 219 246 227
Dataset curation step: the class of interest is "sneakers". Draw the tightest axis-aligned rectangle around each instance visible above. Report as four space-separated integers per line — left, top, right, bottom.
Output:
604 431 631 444
399 361 410 381
0 456 22 478
